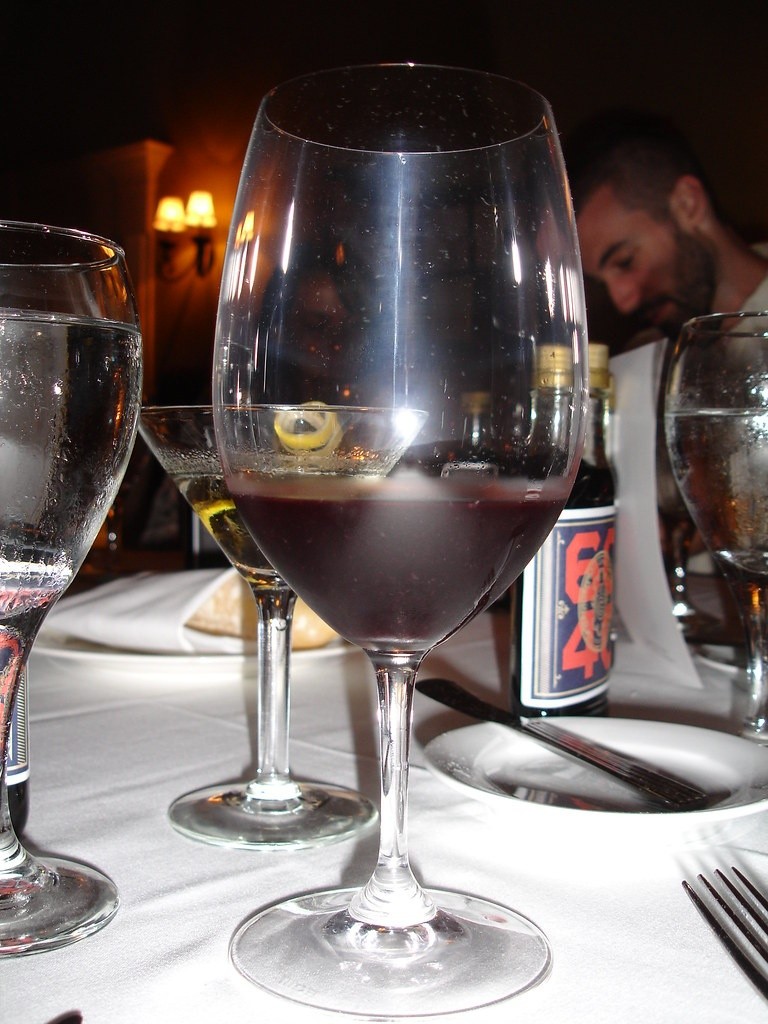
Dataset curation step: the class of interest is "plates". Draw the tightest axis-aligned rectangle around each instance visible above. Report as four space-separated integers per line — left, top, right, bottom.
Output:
426 714 768 812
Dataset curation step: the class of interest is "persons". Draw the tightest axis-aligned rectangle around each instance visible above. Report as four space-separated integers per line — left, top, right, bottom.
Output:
253 254 390 410
522 125 768 552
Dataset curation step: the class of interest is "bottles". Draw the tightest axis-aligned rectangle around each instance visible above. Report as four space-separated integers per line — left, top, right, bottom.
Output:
5 657 30 835
510 342 617 722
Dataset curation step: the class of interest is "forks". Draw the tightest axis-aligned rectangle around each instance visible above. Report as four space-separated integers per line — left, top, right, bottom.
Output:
682 867 768 999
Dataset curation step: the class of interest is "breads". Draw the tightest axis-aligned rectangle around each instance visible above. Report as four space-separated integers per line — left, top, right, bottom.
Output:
186 574 338 649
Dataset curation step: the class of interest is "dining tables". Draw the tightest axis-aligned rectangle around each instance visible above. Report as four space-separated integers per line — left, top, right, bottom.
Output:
0 570 768 1024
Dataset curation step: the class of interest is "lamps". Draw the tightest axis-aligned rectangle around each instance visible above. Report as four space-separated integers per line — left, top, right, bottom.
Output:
184 190 218 277
152 196 194 283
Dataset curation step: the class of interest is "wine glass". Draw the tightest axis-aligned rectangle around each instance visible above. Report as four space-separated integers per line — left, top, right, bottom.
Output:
0 220 142 959
210 66 589 1019
139 403 430 850
663 310 768 744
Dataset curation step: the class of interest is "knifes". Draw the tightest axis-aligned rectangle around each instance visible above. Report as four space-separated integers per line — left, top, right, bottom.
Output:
415 678 708 810
